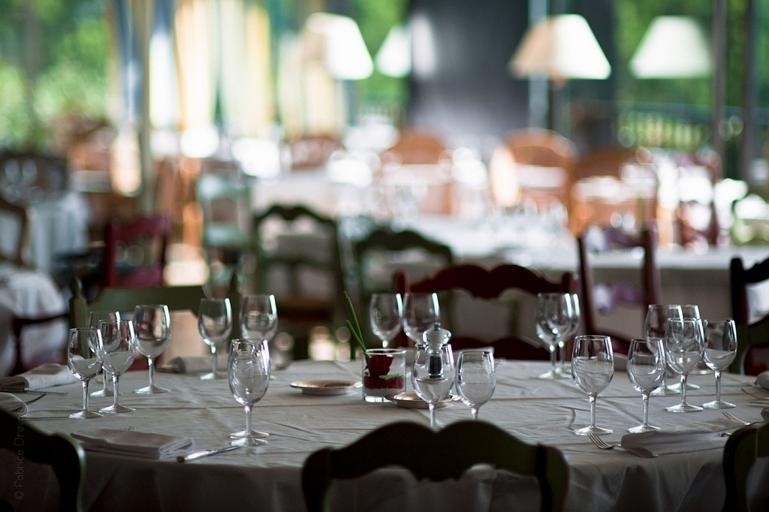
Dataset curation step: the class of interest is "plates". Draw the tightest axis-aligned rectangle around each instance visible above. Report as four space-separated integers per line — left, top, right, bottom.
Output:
386 391 459 410
289 378 363 395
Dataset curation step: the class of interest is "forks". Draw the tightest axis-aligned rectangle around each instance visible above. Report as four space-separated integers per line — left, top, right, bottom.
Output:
586 432 648 458
722 411 766 428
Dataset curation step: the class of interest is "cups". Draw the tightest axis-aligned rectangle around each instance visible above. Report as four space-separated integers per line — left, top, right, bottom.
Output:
362 347 407 403
411 345 496 431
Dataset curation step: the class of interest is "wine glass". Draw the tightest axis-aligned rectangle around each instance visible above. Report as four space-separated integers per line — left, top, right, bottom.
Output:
134 304 172 395
572 333 616 437
66 310 137 419
369 291 441 350
534 293 581 381
626 304 738 433
228 293 279 450
198 297 234 381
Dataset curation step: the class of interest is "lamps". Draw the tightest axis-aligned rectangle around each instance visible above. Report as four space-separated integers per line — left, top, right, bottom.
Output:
296 14 375 132
507 13 612 133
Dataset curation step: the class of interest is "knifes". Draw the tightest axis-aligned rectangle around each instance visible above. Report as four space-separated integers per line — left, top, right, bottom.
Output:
177 445 238 465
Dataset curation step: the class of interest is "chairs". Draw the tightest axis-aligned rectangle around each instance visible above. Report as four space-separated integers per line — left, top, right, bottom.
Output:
0 127 767 508
